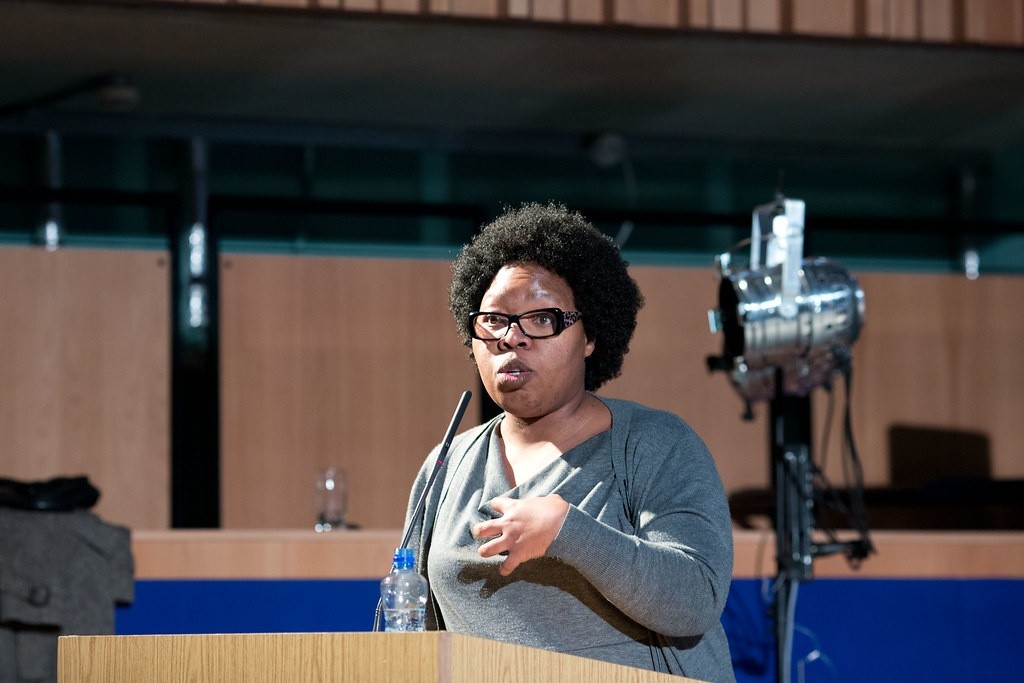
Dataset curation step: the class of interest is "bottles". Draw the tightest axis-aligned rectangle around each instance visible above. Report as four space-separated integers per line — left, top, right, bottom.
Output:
379 548 428 632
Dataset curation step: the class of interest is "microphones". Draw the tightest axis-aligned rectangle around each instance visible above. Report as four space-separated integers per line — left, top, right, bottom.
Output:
372 390 472 633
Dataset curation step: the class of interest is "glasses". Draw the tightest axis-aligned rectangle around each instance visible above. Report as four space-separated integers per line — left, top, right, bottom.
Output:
467 307 582 341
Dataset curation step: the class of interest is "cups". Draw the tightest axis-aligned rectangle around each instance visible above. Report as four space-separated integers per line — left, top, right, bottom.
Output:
315 466 348 529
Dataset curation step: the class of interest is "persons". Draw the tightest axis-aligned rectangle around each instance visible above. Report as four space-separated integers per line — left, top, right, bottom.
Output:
393 205 736 683
